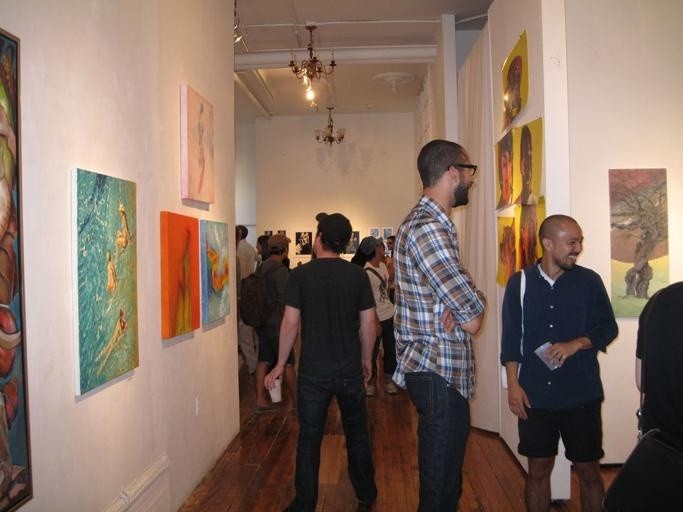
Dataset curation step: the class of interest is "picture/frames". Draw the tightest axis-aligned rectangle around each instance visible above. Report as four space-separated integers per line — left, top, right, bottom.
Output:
0 27 34 511
264 225 394 268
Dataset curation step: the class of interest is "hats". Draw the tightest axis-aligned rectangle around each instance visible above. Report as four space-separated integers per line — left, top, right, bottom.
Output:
316 212 352 241
360 237 383 254
268 234 291 251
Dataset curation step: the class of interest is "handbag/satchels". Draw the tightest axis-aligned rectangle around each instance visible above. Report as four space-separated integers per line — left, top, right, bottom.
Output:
600 427 682 512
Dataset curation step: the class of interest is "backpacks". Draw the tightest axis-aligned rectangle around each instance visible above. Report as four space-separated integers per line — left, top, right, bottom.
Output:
239 263 284 328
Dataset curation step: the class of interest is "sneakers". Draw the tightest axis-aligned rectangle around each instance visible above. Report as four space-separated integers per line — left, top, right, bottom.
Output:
367 385 375 395
385 385 398 393
255 404 279 414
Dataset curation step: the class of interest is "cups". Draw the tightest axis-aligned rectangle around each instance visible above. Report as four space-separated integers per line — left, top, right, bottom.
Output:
533 341 561 374
269 379 283 404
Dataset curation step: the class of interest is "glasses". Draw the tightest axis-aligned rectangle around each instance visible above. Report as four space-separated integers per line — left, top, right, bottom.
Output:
448 163 477 176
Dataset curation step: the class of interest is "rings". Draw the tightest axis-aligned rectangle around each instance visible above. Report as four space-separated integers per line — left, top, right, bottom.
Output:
561 353 567 360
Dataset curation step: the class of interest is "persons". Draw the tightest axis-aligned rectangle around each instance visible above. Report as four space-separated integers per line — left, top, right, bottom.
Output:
104 249 119 295
496 134 514 209
261 210 378 511
632 282 682 450
498 214 620 510
234 224 402 413
94 308 127 375
514 126 538 207
113 201 133 261
387 139 487 512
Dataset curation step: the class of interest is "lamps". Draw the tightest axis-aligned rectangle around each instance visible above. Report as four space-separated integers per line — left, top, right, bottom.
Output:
289 22 338 110
315 103 345 147
233 1 246 51
